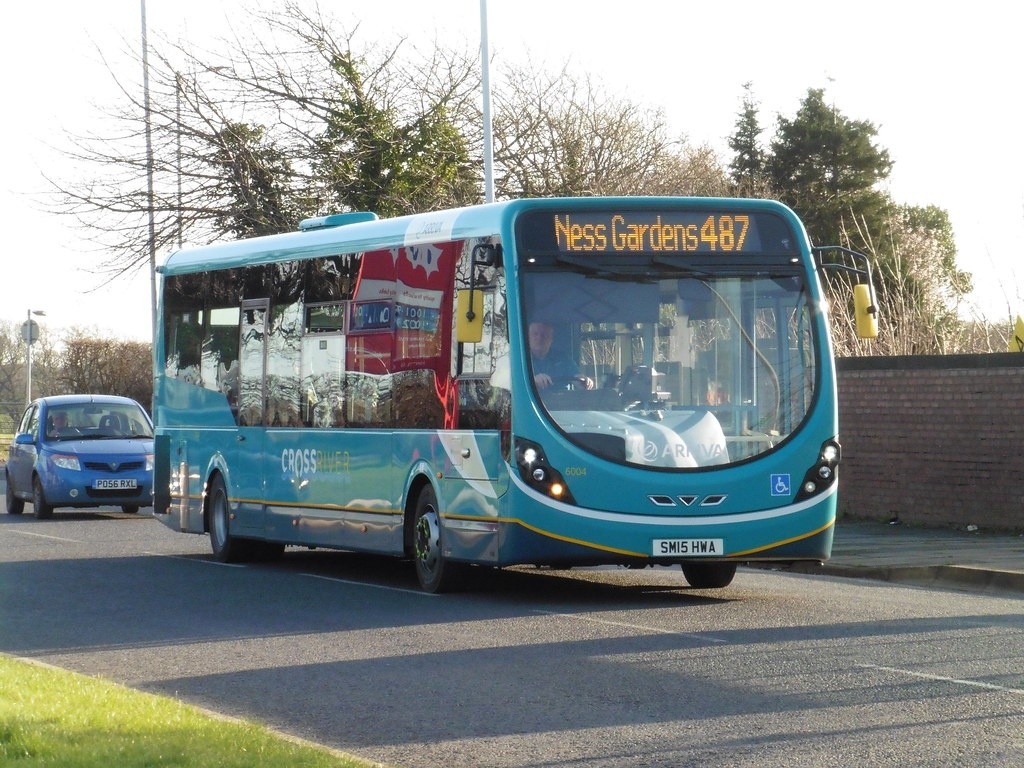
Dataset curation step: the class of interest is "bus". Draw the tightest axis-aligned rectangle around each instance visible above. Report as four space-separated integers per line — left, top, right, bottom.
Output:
149 192 883 594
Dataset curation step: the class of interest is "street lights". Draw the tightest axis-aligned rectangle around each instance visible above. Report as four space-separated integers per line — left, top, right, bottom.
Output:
26 308 45 406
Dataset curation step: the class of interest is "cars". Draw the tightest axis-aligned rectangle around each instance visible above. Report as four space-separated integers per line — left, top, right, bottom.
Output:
5 394 156 519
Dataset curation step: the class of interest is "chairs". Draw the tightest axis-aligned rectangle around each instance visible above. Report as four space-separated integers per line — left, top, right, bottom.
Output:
48 412 68 430
96 415 128 437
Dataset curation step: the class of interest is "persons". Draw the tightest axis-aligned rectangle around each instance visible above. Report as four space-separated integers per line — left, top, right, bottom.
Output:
528 317 594 392
49 411 69 438
